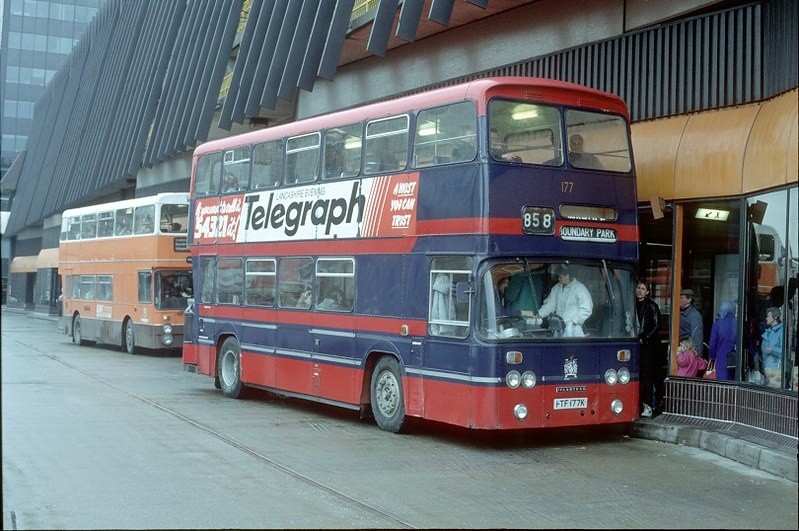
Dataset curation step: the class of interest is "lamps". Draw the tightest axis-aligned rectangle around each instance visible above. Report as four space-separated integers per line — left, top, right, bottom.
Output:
511 108 546 121
343 141 362 150
417 126 446 136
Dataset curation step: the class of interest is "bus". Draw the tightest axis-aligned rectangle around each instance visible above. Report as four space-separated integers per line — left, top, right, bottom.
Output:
59 193 192 354
636 197 799 315
182 76 666 431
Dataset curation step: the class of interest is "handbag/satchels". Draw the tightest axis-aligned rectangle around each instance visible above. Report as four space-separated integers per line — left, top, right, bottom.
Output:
764 358 798 391
703 357 717 380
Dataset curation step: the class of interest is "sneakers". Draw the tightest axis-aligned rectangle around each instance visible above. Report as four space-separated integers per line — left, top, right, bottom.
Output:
640 405 653 417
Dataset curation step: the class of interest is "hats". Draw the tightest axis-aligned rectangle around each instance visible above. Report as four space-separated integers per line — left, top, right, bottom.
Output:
681 288 694 295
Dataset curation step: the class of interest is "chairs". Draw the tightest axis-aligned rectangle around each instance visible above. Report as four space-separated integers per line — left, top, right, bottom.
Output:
292 176 304 184
237 185 247 191
255 180 266 187
431 153 456 164
310 170 320 181
202 189 209 195
273 180 281 187
216 289 233 304
339 167 360 178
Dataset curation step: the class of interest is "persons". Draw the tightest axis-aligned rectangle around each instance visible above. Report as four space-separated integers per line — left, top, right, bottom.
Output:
679 288 703 357
569 133 604 170
184 275 193 295
331 291 349 312
537 264 594 336
479 275 536 337
162 275 178 299
709 300 739 381
677 337 707 378
761 307 784 368
489 128 502 152
635 277 661 418
